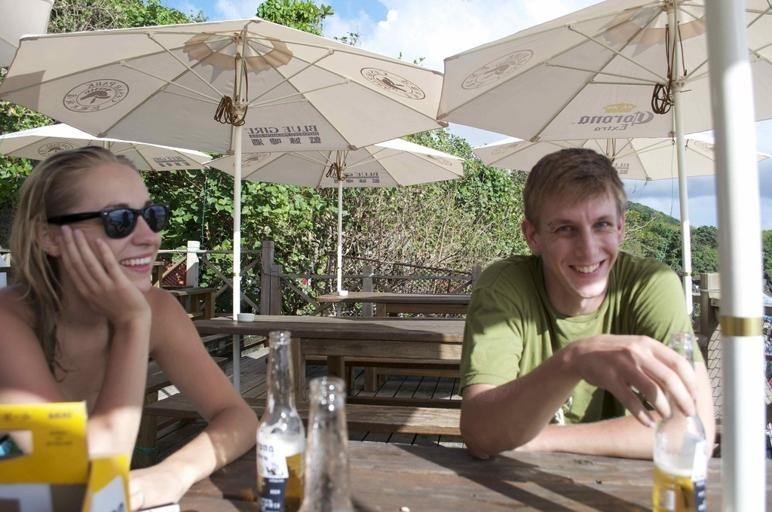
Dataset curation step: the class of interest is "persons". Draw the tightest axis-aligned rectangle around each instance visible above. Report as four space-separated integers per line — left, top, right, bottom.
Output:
1 143 259 512
456 147 716 462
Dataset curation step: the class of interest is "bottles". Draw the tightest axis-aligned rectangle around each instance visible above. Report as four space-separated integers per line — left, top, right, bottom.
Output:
257 330 355 512
655 332 711 512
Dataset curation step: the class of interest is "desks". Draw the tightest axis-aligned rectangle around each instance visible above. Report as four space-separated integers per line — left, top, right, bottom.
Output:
129 287 772 512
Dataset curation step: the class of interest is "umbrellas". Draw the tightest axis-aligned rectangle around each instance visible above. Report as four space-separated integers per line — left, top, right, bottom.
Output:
208 134 466 296
438 2 771 317
469 135 770 183
0 14 447 391
2 0 58 68
0 122 215 172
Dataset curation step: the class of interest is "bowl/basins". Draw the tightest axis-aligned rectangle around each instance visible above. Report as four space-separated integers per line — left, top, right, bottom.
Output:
237 314 255 323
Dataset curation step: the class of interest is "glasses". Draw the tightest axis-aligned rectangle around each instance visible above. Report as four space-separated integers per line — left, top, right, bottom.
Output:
43 204 171 239
